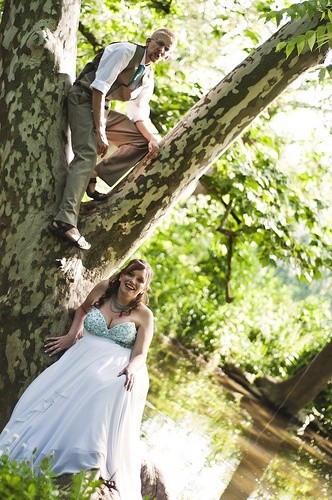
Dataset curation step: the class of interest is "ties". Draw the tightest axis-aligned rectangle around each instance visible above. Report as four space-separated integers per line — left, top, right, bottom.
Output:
129 64 146 89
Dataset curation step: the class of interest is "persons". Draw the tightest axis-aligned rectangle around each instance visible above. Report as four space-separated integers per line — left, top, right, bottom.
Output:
0 256 156 498
46 27 175 249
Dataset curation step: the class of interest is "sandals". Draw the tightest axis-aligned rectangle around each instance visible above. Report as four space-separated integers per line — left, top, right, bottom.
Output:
85 175 104 199
49 219 92 251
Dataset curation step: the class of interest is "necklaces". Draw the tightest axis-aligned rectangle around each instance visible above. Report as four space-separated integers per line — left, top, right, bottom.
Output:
109 293 131 314
